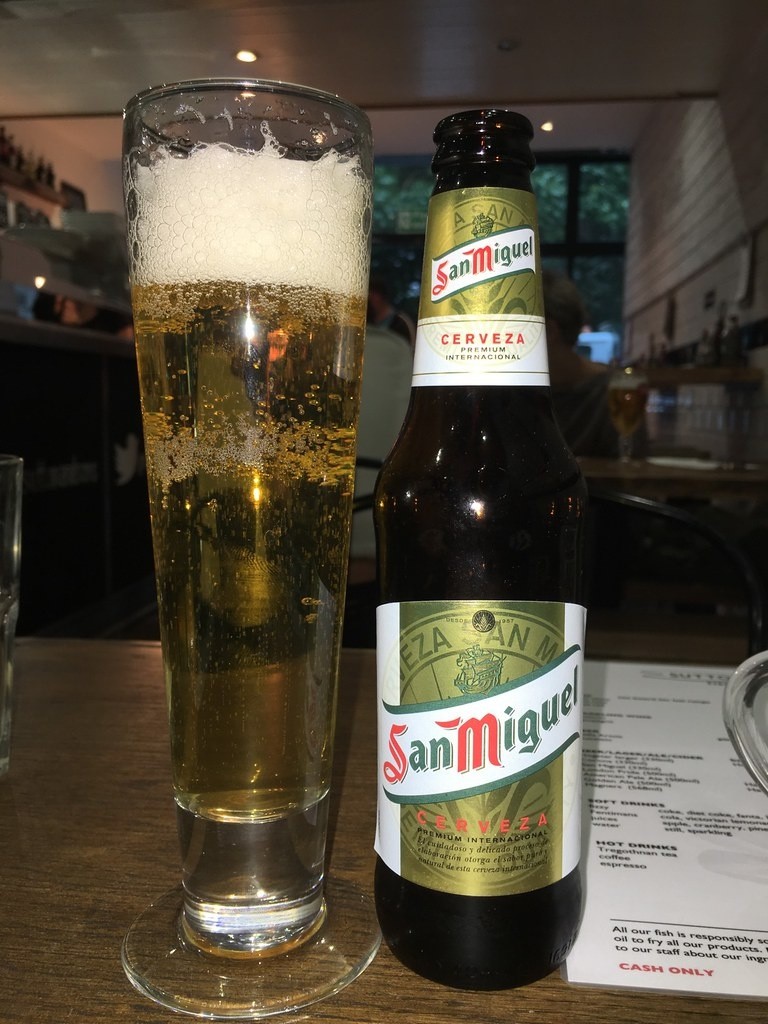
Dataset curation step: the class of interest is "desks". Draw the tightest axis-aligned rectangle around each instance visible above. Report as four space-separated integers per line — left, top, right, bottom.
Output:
564 446 768 618
0 637 767 1023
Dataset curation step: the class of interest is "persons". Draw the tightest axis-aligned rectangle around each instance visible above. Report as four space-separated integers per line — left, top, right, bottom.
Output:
27 284 134 342
546 284 624 460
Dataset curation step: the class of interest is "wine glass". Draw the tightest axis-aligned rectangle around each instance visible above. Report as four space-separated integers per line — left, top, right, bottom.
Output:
608 370 646 466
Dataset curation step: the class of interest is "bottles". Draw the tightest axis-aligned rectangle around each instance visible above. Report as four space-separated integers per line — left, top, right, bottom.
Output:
372 108 601 990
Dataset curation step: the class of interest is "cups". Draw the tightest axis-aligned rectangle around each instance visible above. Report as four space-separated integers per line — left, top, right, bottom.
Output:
0 450 22 777
724 651 768 800
116 78 385 1017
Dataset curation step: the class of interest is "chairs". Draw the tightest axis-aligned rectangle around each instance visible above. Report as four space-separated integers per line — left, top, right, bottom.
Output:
341 478 764 668
